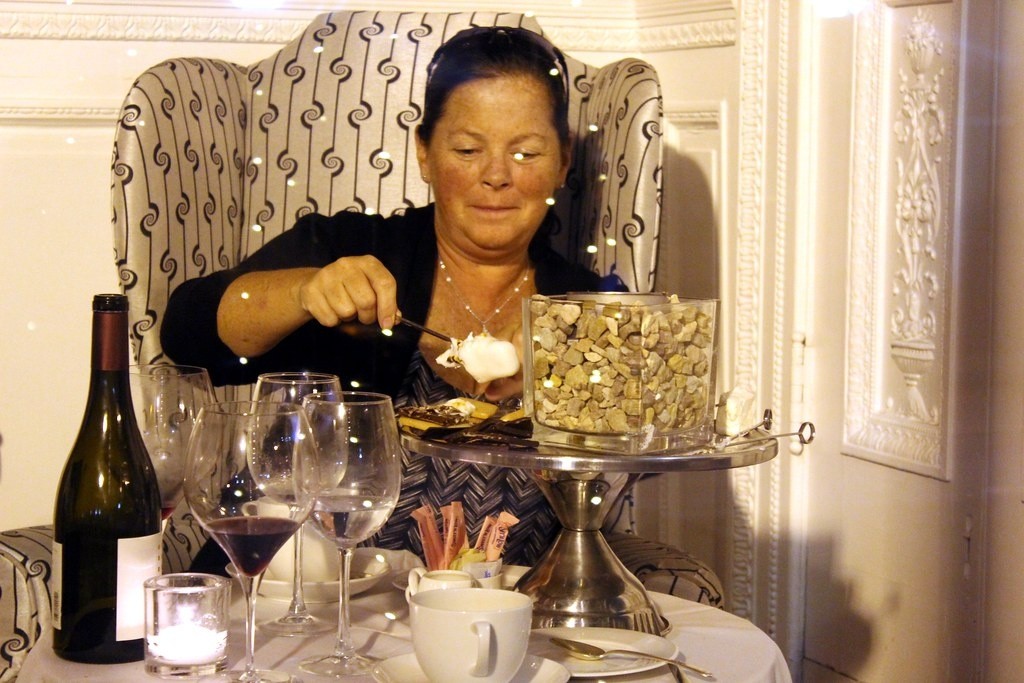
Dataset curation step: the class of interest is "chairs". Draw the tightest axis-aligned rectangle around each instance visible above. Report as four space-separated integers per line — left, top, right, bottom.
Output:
1 8 724 682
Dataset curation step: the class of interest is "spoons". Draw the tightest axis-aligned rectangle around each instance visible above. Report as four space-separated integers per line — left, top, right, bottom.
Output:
547 637 713 680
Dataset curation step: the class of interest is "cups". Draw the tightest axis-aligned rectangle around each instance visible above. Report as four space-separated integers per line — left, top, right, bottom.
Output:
143 572 233 681
407 568 472 595
410 587 534 683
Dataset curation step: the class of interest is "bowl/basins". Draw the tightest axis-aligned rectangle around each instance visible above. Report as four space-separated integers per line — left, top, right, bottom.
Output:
520 290 721 456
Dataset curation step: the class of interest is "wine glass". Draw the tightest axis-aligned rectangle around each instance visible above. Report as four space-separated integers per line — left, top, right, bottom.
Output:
126 363 224 536
290 390 402 679
244 373 349 638
181 400 320 683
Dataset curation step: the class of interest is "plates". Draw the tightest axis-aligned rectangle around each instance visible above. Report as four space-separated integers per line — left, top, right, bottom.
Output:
371 652 572 683
224 547 393 604
529 627 680 678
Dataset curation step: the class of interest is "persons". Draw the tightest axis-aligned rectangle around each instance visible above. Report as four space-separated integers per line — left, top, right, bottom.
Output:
162 26 629 588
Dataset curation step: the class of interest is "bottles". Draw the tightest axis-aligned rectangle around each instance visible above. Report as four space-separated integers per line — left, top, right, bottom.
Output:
49 294 166 664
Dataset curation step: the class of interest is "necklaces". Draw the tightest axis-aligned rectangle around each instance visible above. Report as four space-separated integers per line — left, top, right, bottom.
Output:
437 256 530 339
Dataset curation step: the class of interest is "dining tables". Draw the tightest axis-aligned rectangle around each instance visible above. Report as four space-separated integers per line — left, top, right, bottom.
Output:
9 565 794 683
